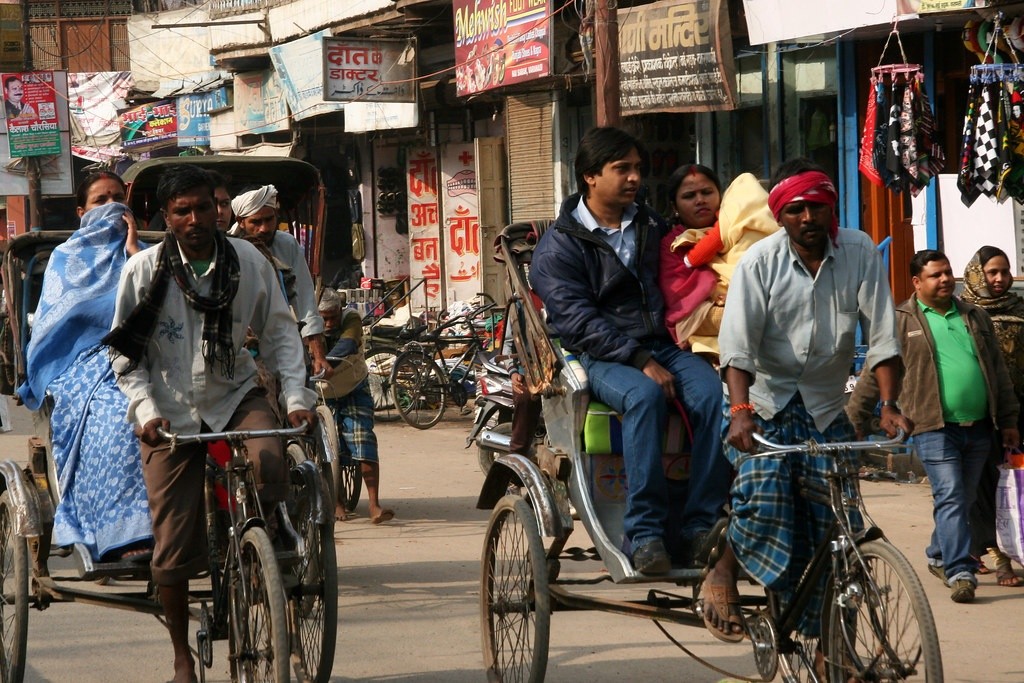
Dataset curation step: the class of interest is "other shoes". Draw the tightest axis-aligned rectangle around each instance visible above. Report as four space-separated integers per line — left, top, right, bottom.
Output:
977 563 990 573
691 518 728 568
634 540 670 573
994 557 1023 585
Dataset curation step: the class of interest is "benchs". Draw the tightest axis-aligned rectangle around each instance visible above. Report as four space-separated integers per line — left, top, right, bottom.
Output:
492 217 686 458
2 228 165 447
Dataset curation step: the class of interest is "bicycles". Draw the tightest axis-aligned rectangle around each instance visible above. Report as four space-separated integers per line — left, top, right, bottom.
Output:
364 290 500 430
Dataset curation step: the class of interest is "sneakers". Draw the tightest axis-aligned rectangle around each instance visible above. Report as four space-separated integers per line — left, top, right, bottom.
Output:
928 564 950 587
951 579 975 602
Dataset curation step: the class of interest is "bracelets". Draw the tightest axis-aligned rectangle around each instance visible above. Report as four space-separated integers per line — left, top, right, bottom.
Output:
881 401 899 410
730 405 755 417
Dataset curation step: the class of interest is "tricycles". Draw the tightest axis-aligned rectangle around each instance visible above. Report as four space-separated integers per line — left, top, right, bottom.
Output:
0 156 364 683
463 218 944 683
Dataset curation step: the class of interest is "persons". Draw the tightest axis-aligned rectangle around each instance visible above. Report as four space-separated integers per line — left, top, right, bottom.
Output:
109 167 336 683
846 244 1024 604
306 289 395 525
503 311 542 496
529 125 783 574
4 77 37 119
67 74 80 88
699 157 915 683
26 172 157 565
112 72 132 99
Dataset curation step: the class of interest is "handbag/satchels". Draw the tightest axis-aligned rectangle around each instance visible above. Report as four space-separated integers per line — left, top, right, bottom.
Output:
995 445 1024 566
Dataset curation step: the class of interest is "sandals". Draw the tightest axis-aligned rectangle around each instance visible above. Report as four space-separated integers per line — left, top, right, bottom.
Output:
704 570 746 642
